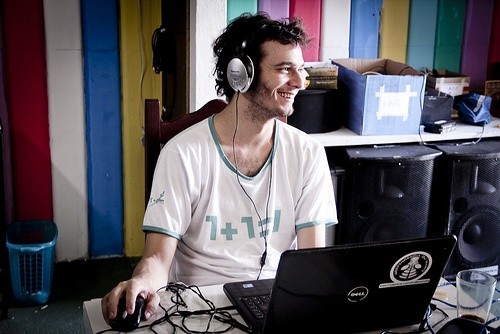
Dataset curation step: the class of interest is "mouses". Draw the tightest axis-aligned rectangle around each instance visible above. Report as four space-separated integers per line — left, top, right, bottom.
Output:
109 296 146 333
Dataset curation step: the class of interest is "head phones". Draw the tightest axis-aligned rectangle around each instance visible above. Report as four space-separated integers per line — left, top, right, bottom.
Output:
227 21 267 94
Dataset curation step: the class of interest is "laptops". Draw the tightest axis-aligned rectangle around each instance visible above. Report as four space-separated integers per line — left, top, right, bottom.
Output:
224 236 458 334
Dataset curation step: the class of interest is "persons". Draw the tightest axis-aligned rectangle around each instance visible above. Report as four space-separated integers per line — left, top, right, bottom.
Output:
100 10 338 323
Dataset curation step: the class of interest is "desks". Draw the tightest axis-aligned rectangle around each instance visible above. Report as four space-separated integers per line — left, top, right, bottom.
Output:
84 269 500 334
310 115 500 147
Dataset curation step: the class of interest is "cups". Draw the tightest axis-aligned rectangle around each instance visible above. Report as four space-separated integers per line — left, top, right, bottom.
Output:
456 270 497 325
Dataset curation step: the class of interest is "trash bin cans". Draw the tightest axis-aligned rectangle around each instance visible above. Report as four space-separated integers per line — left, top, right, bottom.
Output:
5 219 59 306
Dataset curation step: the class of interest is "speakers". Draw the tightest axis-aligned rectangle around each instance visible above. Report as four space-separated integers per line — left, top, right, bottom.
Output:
337 137 500 282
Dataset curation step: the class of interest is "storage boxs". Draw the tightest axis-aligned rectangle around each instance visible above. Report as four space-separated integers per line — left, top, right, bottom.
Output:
329 59 426 135
425 68 470 117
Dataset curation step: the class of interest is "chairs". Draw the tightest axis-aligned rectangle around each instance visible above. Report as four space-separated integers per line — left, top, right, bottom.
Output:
145 96 228 212
